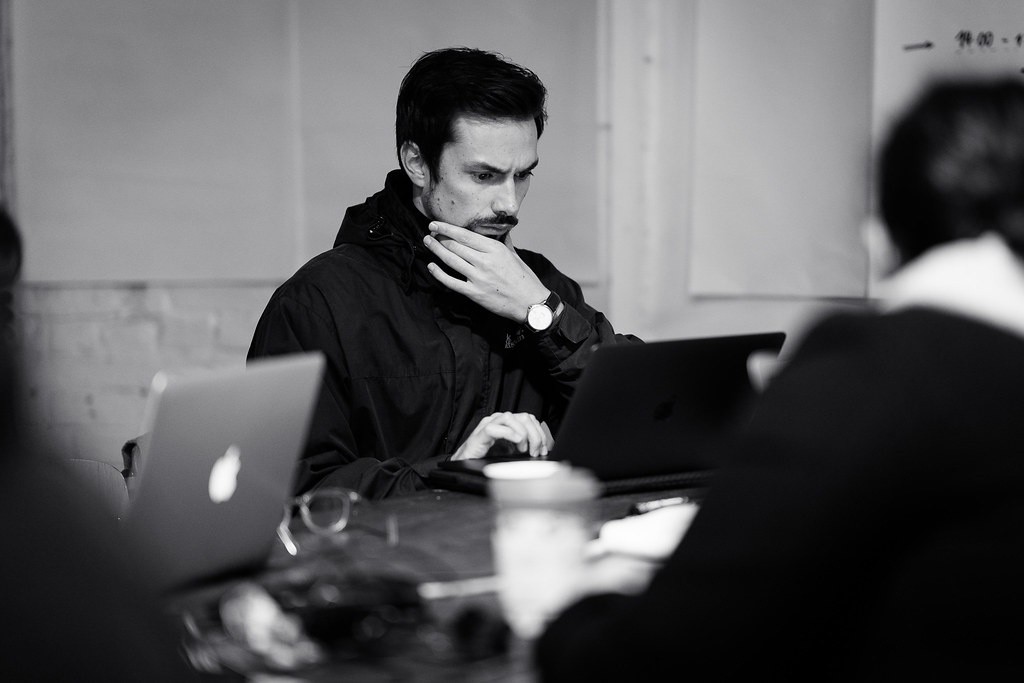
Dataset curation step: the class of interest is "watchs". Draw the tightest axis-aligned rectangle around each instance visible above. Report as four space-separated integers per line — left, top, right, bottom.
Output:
522 290 562 337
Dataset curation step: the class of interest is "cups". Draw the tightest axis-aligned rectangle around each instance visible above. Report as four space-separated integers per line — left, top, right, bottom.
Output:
482 461 598 635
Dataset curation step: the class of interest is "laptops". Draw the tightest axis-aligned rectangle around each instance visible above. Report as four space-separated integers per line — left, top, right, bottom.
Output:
437 330 788 485
128 354 324 599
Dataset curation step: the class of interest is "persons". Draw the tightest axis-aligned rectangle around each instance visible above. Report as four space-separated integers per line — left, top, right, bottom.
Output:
245 45 646 519
528 69 1024 682
